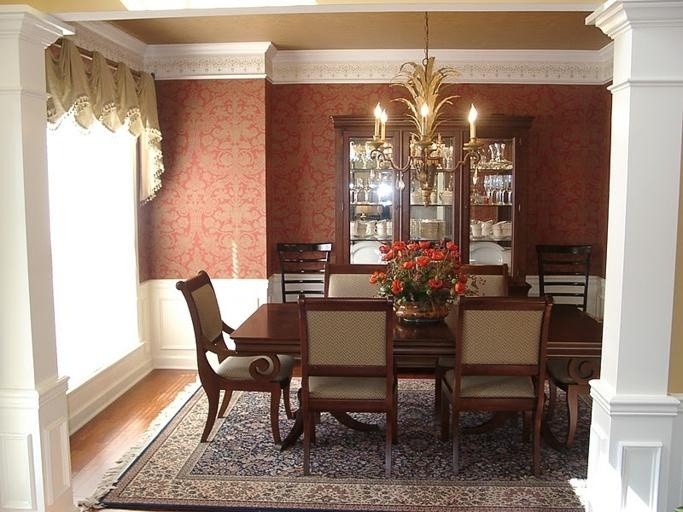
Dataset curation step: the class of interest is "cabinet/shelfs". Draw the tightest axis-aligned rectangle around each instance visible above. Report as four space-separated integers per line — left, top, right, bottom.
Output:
329 114 535 285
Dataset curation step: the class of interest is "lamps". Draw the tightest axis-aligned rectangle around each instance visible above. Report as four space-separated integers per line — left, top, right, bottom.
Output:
370 12 484 208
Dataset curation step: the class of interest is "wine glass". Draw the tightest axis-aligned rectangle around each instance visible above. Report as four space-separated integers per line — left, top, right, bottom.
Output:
351 178 384 204
484 175 512 205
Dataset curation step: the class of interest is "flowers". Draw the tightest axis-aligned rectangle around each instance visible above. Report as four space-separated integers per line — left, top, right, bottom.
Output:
369 239 486 311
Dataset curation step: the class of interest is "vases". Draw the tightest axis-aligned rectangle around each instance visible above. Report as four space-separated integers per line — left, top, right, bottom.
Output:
393 299 451 326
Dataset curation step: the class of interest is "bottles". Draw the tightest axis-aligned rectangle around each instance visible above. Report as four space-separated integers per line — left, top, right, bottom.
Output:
488 143 505 163
441 190 453 205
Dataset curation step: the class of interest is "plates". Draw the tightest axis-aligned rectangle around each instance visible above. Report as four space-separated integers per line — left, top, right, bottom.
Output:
350 240 389 264
470 240 505 265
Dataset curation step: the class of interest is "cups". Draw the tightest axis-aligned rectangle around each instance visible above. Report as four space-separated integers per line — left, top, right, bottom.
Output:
470 222 512 237
350 220 392 237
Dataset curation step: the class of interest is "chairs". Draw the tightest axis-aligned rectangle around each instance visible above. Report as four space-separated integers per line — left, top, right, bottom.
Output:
546 359 600 448
276 242 333 303
176 270 293 445
459 264 509 297
440 295 554 479
534 245 592 311
298 299 398 478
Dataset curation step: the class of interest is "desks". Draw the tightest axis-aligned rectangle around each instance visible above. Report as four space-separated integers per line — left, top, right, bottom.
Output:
230 303 603 452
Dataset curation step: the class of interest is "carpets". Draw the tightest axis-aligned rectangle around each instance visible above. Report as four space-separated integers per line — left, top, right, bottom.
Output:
78 384 593 512
